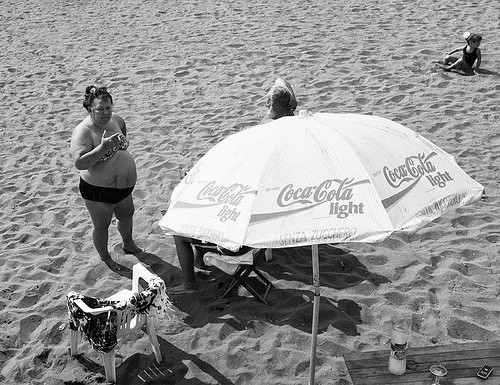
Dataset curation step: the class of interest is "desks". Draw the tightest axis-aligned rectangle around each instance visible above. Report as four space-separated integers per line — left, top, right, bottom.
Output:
342 341 500 385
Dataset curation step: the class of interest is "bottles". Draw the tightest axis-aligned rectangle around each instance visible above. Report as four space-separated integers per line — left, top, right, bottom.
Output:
388 317 408 375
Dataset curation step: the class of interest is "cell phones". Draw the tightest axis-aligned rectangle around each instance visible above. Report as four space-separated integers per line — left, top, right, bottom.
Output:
477 365 493 380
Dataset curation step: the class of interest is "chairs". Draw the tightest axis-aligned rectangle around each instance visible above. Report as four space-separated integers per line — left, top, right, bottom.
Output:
66 263 164 382
222 248 275 305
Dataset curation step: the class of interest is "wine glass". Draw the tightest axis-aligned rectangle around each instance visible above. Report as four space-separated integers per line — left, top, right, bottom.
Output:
429 365 447 385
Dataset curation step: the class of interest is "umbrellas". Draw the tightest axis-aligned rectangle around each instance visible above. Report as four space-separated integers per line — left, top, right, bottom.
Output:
158 109 485 385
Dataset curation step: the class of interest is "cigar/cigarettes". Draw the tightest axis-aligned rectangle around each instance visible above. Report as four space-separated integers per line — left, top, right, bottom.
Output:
117 135 120 141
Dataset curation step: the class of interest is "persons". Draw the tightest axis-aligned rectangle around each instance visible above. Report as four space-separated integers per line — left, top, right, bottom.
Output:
435 29 484 74
263 76 299 120
70 85 143 271
169 233 254 295
